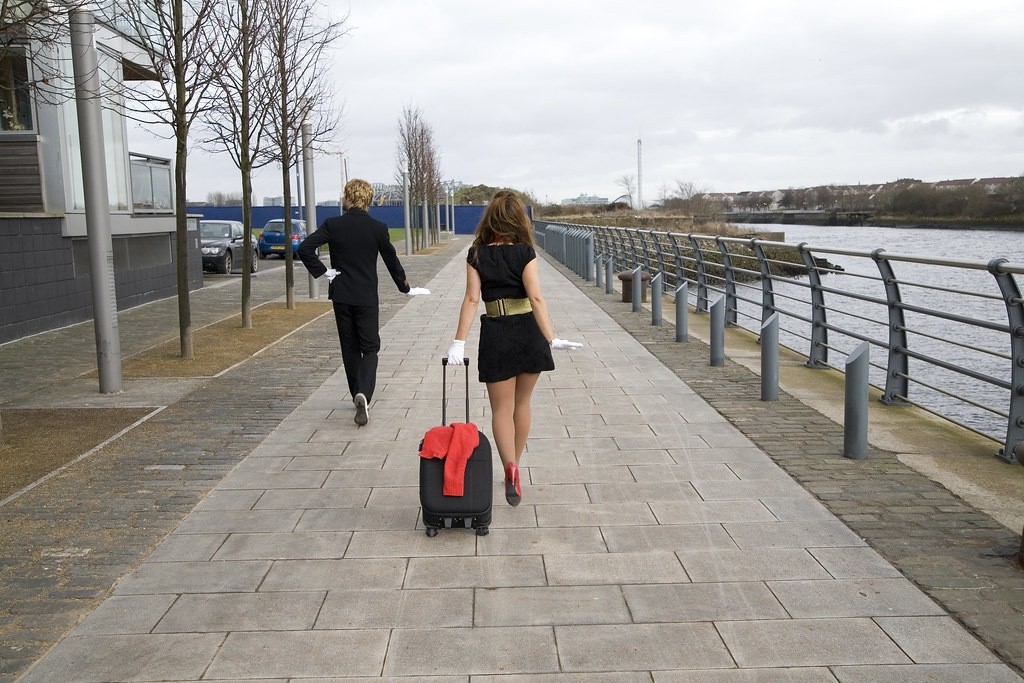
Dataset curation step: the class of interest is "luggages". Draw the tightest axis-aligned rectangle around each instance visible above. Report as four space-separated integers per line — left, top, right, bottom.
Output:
418 357 493 537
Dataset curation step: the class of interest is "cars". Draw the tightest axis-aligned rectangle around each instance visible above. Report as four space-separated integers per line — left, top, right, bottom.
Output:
258 219 319 260
199 219 260 274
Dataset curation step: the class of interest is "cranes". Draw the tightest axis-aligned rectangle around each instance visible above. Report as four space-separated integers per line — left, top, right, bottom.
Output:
638 139 642 210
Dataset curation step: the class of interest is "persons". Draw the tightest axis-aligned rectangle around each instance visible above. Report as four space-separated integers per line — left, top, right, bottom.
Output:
297 180 432 425
448 191 584 507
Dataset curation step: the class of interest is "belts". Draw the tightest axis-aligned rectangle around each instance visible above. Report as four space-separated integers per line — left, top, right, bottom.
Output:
485 297 533 318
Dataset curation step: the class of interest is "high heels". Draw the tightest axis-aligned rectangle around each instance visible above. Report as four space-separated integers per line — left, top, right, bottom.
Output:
505 462 522 507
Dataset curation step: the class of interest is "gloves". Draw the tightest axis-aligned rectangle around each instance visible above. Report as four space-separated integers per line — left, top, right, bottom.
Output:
447 340 466 366
322 267 341 284
550 338 583 353
406 286 431 296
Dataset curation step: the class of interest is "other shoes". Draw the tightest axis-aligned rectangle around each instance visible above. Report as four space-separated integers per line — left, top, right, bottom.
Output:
354 393 369 425
